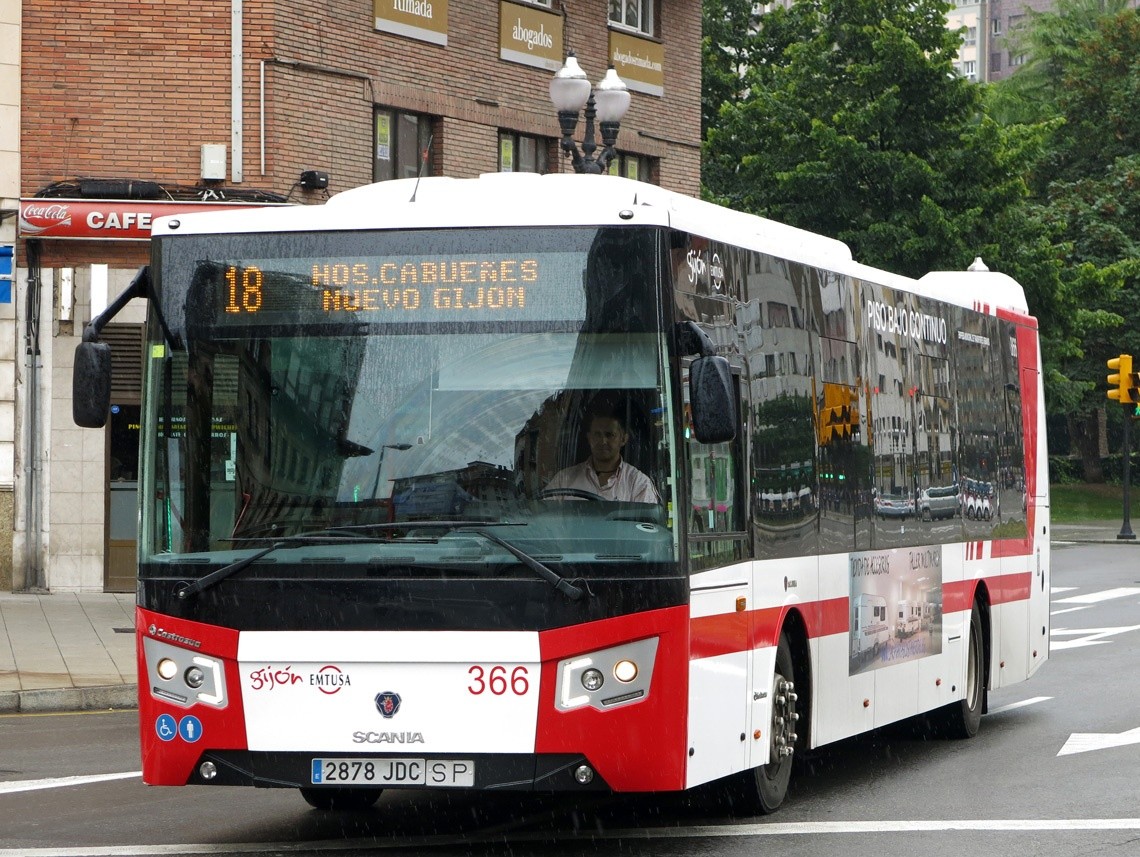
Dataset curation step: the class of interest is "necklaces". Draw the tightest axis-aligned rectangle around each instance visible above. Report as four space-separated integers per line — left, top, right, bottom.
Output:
596 469 617 475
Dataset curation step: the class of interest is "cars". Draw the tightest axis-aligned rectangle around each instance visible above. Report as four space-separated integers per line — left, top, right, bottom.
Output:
874 486 959 521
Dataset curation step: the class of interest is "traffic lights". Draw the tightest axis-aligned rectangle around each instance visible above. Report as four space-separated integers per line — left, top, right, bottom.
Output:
1127 372 1140 418
1107 355 1134 403
813 377 863 446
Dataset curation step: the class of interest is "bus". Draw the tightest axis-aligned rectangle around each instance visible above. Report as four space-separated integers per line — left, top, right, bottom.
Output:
961 476 995 521
72 171 1051 813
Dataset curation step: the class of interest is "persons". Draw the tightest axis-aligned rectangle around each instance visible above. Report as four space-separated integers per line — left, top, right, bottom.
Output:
541 409 663 505
240 414 337 499
